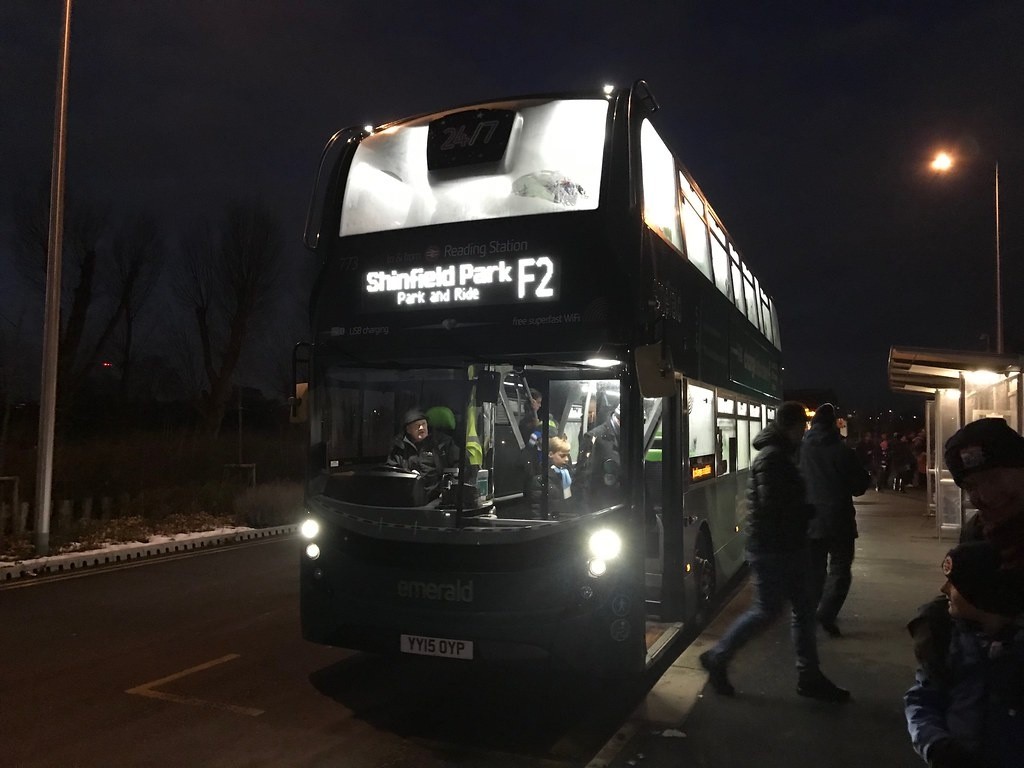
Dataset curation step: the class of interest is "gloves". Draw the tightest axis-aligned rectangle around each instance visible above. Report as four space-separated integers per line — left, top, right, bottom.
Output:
527 431 542 447
927 739 974 768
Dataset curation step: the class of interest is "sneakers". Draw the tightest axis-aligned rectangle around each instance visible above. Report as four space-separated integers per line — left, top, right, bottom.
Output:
699 649 737 698
797 671 851 698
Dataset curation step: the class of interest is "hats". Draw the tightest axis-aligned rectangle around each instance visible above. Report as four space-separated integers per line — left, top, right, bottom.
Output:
402 410 429 427
811 404 837 424
614 403 646 424
777 401 812 423
943 417 1024 487
942 542 1024 615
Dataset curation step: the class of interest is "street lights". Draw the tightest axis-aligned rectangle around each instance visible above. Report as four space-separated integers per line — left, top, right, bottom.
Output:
928 148 1003 356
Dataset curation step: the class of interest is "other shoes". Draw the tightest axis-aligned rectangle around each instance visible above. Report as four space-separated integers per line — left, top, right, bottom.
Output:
816 602 841 637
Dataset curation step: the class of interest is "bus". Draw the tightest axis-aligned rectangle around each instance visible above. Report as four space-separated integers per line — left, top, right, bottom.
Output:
290 79 788 681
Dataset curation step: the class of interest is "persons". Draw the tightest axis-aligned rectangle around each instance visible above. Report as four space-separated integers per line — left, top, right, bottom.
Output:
903 418 1024 768
856 429 927 492
699 401 870 703
387 408 460 501
476 389 620 518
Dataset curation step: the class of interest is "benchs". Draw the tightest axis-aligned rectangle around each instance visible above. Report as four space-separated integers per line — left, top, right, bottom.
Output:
927 468 978 518
